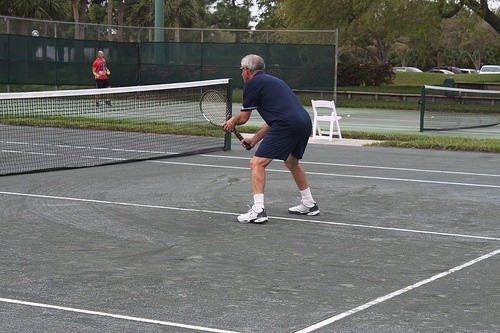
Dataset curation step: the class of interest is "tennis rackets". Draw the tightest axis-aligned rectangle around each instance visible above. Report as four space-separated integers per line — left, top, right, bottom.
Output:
200 90 251 151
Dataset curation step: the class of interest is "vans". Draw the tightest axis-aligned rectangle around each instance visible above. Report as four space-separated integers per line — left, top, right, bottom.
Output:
391 66 426 73
460 68 476 75
443 67 463 74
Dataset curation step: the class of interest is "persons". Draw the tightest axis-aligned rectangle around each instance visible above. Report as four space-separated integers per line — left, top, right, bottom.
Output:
222 54 320 224
92 50 113 107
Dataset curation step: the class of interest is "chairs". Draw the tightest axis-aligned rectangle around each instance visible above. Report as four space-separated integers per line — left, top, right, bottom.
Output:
311 100 343 141
443 77 459 102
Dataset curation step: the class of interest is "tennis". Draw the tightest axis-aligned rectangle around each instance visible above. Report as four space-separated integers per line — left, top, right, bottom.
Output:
346 114 351 118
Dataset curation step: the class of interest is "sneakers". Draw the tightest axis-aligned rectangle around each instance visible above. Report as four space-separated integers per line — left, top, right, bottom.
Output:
237 205 269 223
288 197 320 216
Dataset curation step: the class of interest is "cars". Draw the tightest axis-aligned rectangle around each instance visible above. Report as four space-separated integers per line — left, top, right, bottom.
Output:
426 68 455 76
478 65 500 75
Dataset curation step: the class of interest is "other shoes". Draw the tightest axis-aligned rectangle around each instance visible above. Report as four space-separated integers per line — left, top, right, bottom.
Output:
104 101 112 106
96 102 99 107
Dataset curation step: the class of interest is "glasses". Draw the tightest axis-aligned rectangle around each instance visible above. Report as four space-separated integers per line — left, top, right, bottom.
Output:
240 67 246 73
